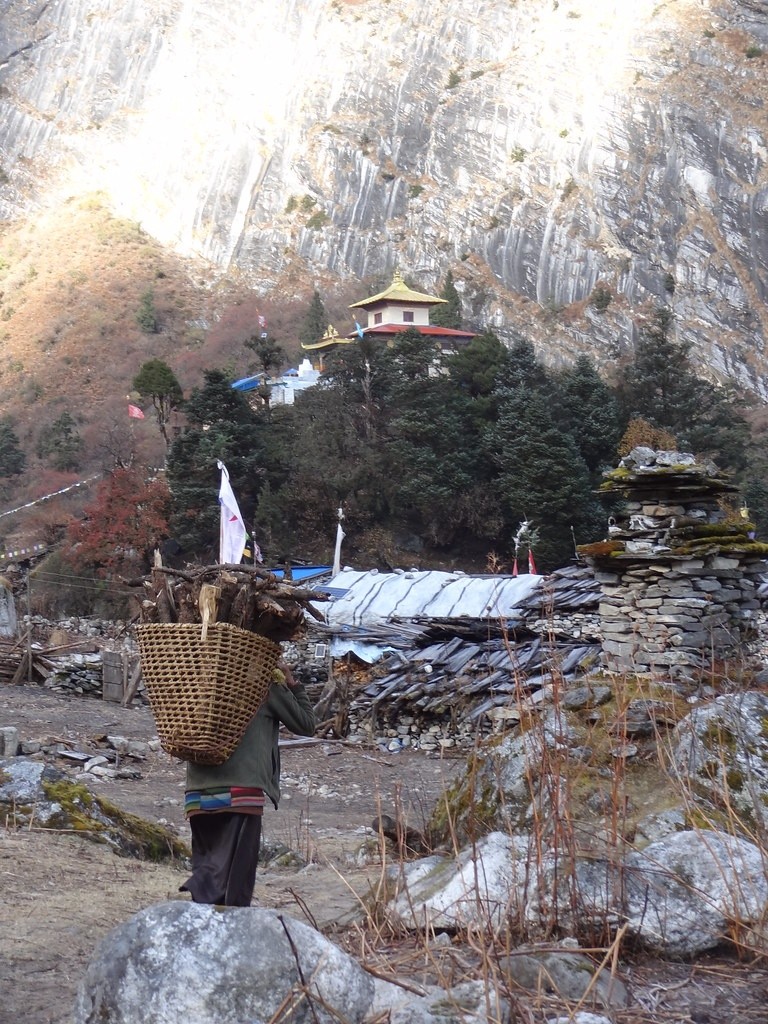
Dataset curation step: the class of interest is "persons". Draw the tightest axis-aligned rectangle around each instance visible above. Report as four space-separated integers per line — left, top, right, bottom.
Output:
179 664 316 907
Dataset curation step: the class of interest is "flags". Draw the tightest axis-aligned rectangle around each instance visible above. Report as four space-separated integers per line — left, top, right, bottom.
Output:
529 550 537 574
258 315 266 329
221 474 247 564
128 404 145 419
355 324 364 337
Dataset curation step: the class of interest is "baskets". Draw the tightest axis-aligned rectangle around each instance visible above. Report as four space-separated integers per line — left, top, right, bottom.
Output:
135 621 285 764
